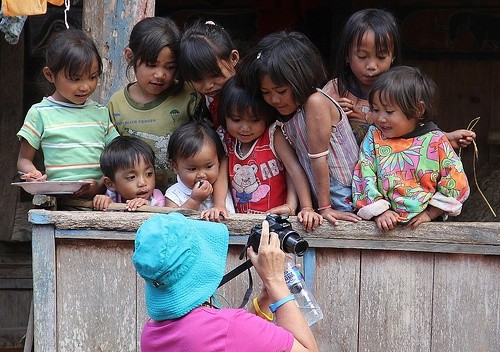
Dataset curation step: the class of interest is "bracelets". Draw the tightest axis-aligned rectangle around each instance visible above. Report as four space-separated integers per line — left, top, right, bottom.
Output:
316 205 332 210
307 150 329 158
253 298 272 322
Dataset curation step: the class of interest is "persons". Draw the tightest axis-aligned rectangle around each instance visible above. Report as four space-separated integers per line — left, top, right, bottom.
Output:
132 212 319 352
17 8 475 232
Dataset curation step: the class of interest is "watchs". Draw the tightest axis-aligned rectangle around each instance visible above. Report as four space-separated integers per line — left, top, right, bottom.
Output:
269 295 295 313
283 204 294 215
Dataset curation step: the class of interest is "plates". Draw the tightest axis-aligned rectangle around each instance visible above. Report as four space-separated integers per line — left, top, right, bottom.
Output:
11 181 93 195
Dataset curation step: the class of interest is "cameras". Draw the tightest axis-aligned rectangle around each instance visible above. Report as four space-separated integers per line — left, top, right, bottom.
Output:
247 213 309 257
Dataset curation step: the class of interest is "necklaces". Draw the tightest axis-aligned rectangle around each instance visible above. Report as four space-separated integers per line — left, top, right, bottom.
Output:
237 140 245 158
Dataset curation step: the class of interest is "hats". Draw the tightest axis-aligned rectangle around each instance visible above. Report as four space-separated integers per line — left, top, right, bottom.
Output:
132 212 229 320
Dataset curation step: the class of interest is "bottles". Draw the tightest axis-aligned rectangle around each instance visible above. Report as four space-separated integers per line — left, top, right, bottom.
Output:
284 257 324 327
